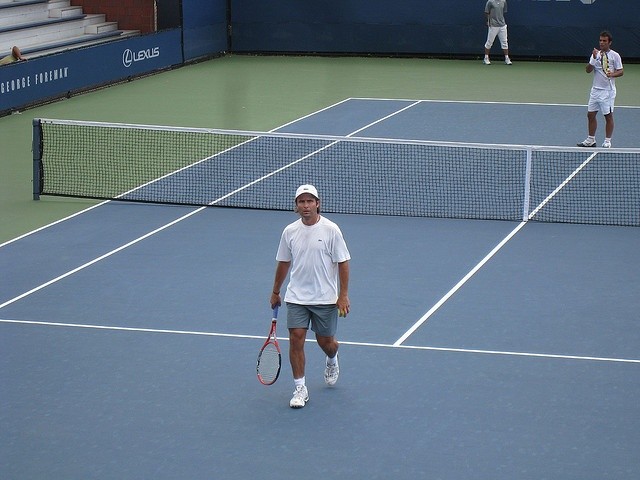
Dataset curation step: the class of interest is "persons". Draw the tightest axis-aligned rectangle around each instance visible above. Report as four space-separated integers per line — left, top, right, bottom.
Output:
270 181 351 409
579 32 625 148
484 0 518 65
0 43 31 65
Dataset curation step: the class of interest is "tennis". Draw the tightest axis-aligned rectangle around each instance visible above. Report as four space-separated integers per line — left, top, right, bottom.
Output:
338 309 345 317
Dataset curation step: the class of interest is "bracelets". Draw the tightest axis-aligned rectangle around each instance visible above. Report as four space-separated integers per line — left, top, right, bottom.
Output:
589 57 597 65
273 291 280 294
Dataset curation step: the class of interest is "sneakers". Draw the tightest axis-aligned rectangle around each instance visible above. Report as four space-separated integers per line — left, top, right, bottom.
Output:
576 140 597 148
504 58 512 65
324 352 339 386
482 57 491 64
289 383 310 408
600 141 612 148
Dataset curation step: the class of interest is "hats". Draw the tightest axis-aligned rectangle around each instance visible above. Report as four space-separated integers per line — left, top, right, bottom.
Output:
294 183 319 200
